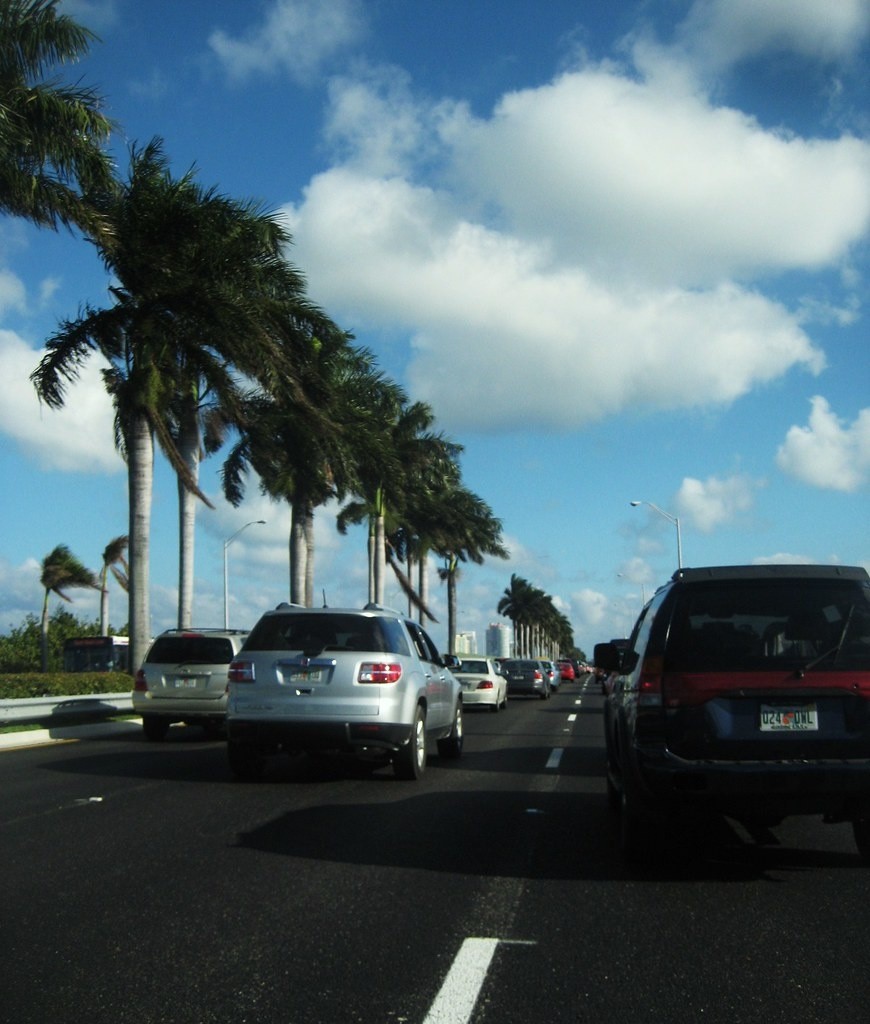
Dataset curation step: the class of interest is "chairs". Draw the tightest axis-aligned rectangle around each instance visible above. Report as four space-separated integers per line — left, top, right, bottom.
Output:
687 620 840 670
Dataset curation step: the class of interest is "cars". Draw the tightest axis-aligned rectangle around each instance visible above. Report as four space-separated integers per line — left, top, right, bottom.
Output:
502 660 549 699
542 659 591 692
448 659 509 712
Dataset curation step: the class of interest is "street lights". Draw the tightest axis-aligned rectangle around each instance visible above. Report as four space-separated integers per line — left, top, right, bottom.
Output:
631 500 683 565
224 521 266 628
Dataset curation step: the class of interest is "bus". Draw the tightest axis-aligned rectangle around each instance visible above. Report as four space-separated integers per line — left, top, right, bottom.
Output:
55 636 156 671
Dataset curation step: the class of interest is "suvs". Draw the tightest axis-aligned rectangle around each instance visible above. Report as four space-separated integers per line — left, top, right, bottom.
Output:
226 602 465 780
132 629 252 738
593 566 870 858
